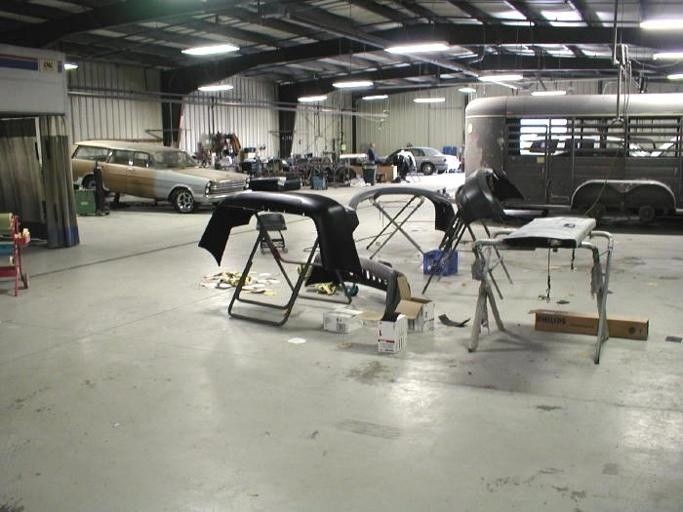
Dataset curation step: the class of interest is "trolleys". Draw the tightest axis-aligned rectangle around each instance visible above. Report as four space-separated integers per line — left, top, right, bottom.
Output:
256 214 289 255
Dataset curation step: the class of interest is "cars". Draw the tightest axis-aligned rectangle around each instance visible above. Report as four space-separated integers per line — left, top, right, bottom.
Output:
65 137 251 215
338 153 390 182
383 145 461 175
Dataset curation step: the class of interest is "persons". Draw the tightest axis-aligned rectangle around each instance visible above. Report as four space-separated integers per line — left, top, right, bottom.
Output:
398 155 413 176
219 149 232 167
368 143 376 165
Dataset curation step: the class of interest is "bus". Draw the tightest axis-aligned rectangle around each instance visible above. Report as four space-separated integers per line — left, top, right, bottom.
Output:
459 89 682 225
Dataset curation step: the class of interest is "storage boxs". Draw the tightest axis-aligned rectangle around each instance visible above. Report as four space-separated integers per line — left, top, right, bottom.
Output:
527 309 649 341
376 314 408 353
423 250 458 275
323 307 364 334
394 276 435 333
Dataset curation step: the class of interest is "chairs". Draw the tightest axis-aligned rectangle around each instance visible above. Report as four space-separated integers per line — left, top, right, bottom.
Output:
257 214 288 250
